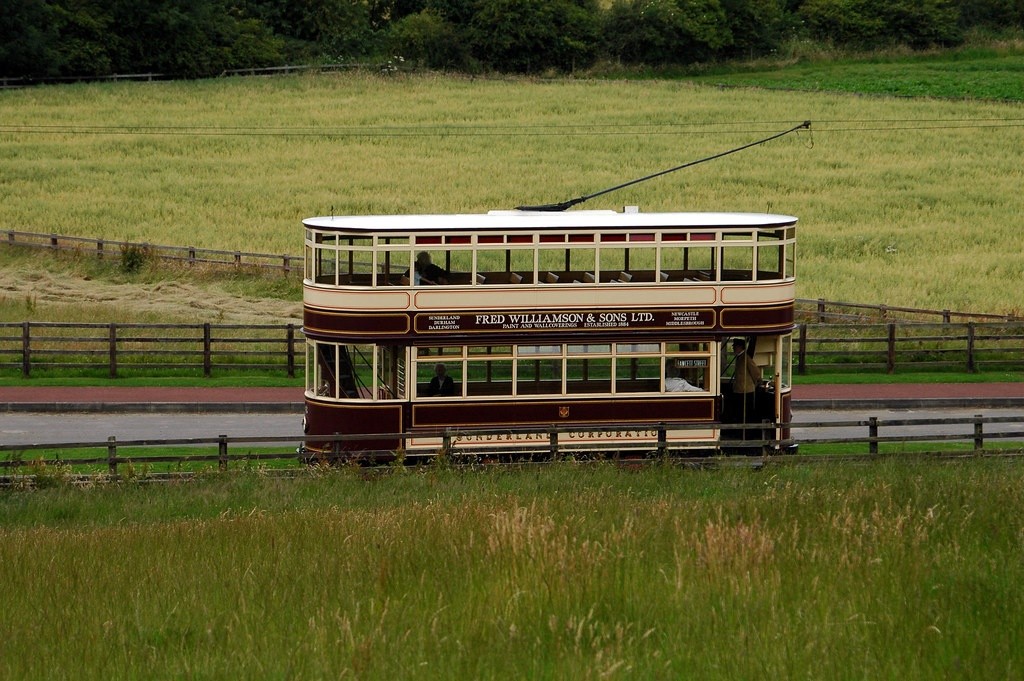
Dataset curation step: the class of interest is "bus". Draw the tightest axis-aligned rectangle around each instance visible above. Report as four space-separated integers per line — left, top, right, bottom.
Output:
299 209 802 468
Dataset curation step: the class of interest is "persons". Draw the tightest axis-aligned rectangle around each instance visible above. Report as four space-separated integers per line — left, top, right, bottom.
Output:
426 364 455 397
731 339 760 418
665 360 706 392
403 251 449 283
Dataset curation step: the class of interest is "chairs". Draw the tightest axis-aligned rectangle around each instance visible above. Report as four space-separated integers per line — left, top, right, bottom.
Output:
400 271 713 285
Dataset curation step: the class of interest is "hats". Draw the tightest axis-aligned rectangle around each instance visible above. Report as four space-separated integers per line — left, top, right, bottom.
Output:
732 338 746 349
